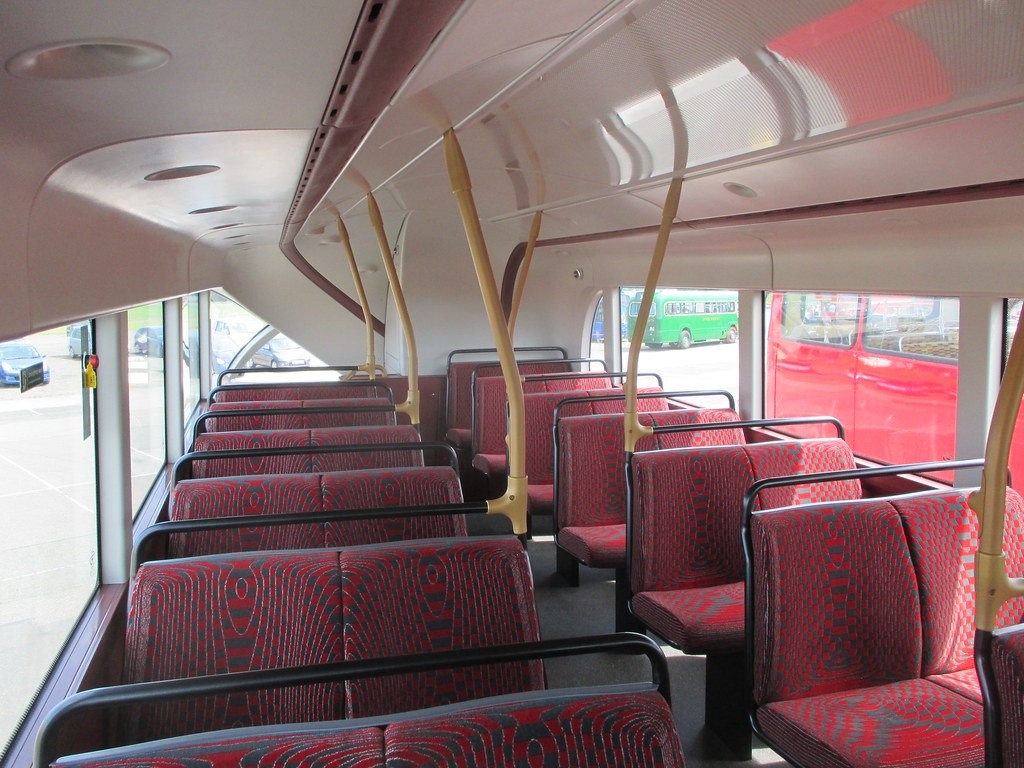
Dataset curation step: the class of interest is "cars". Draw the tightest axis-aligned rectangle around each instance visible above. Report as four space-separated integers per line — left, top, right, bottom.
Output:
182 332 241 372
134 327 165 358
253 338 311 368
70 327 95 358
1 341 50 386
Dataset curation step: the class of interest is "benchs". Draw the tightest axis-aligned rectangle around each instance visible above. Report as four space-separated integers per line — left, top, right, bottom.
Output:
190 404 424 477
117 498 546 747
444 348 574 455
212 366 379 397
38 632 683 768
507 372 669 541
549 390 747 585
207 384 398 431
165 442 472 552
741 460 1024 768
471 360 614 500
625 419 865 760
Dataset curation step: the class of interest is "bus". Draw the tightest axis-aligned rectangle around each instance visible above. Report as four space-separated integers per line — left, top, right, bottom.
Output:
767 290 1024 500
626 289 738 349
